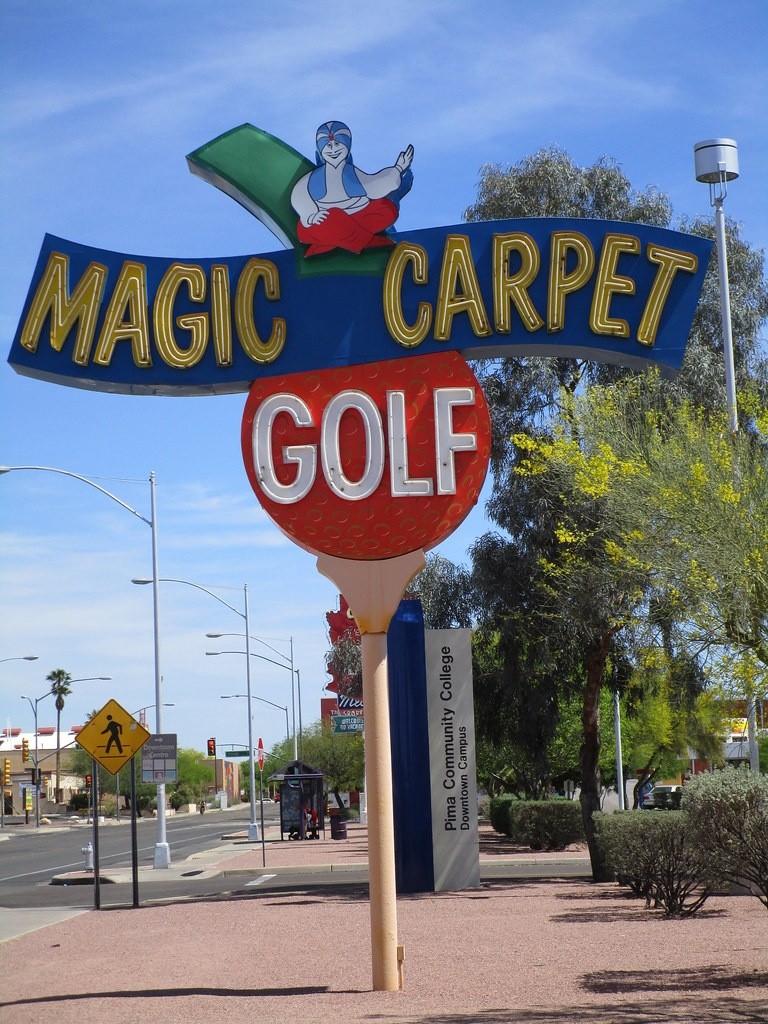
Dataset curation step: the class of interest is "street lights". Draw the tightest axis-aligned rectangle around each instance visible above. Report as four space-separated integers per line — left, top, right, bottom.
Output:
695 139 763 784
21 676 112 827
204 633 303 781
131 577 259 841
0 465 173 871
93 703 176 822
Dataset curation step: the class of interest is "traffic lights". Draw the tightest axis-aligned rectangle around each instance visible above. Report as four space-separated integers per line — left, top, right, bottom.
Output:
3 758 11 785
23 739 29 763
45 778 48 787
86 775 92 788
208 739 216 757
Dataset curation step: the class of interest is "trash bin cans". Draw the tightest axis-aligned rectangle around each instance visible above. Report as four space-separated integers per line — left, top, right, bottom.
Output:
330 815 347 840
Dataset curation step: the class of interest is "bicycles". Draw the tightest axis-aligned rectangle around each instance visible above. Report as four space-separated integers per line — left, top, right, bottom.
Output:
199 804 205 814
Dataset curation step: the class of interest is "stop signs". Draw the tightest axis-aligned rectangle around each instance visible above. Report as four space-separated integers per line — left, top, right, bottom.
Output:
258 737 266 772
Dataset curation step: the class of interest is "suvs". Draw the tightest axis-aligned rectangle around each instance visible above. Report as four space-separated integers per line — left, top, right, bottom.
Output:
274 795 281 803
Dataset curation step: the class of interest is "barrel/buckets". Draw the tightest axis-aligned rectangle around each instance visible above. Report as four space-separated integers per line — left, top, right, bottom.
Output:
330 815 346 840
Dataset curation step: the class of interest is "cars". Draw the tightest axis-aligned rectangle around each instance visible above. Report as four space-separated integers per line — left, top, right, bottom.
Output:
644 786 683 809
